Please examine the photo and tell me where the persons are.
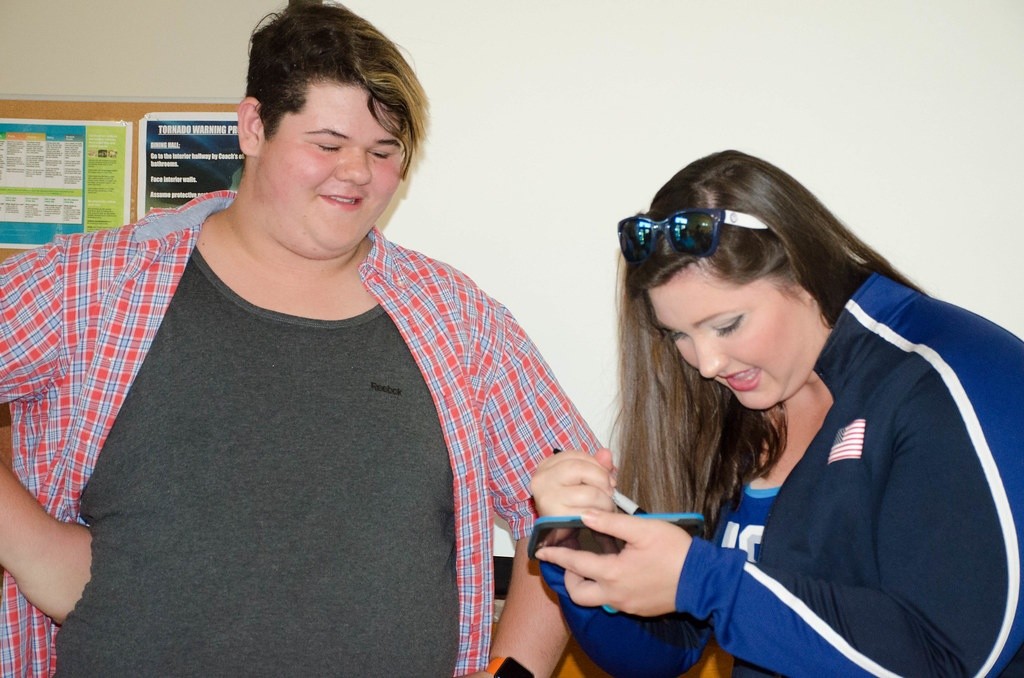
[531,151,1024,678]
[0,0,617,678]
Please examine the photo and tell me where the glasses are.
[618,210,769,263]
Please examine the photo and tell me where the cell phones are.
[526,512,704,562]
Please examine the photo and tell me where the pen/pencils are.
[551,448,647,515]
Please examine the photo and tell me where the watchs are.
[486,656,534,678]
